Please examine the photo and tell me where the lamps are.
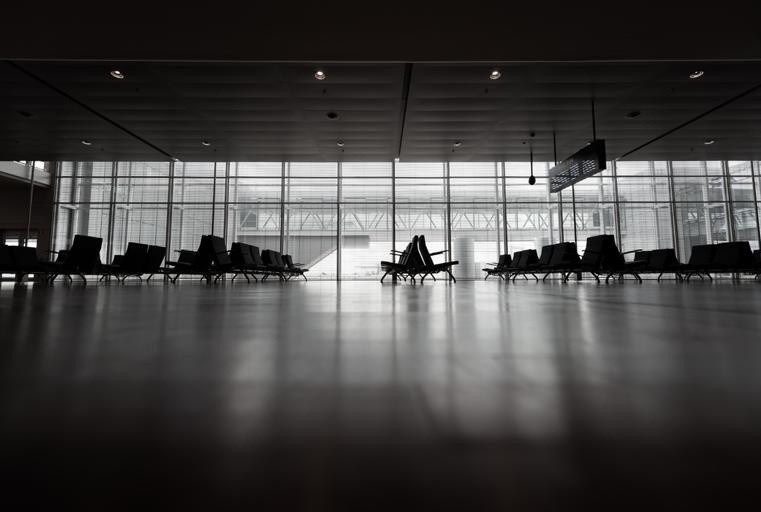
[312,67,328,81]
[687,62,705,79]
[703,132,720,145]
[486,65,505,83]
[109,63,128,81]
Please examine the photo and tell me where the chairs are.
[482,235,760,287]
[21,234,309,289]
[380,235,458,284]
